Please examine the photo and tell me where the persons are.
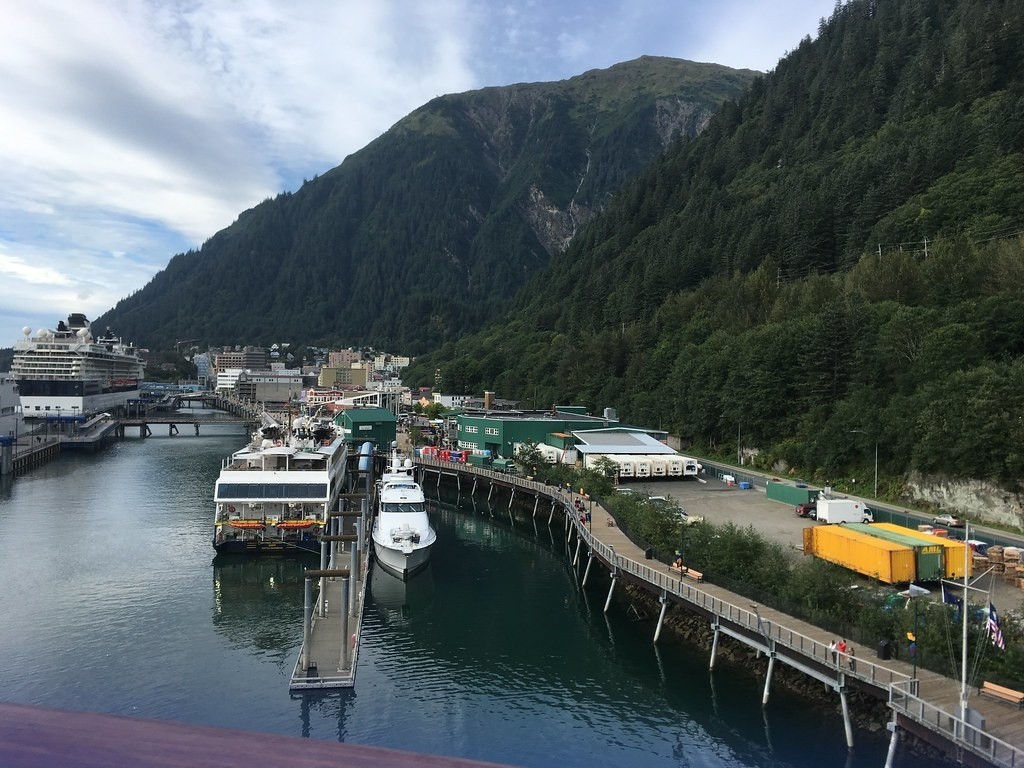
[574,496,585,525]
[829,640,837,664]
[837,639,846,664]
[847,647,855,671]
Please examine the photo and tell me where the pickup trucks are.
[796,503,818,520]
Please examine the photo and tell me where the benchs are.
[668,562,704,583]
[977,681,1024,711]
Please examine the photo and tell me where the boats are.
[211,408,350,560]
[370,558,438,621]
[110,379,125,387]
[371,439,437,574]
[126,380,138,387]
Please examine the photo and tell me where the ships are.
[8,313,148,419]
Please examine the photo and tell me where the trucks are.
[817,499,875,526]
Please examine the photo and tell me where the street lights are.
[850,430,878,499]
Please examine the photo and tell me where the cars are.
[933,514,965,528]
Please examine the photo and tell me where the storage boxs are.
[724,474,735,487]
[416,445,472,464]
[738,482,751,489]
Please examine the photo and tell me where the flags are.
[944,588,962,605]
[988,601,1005,650]
